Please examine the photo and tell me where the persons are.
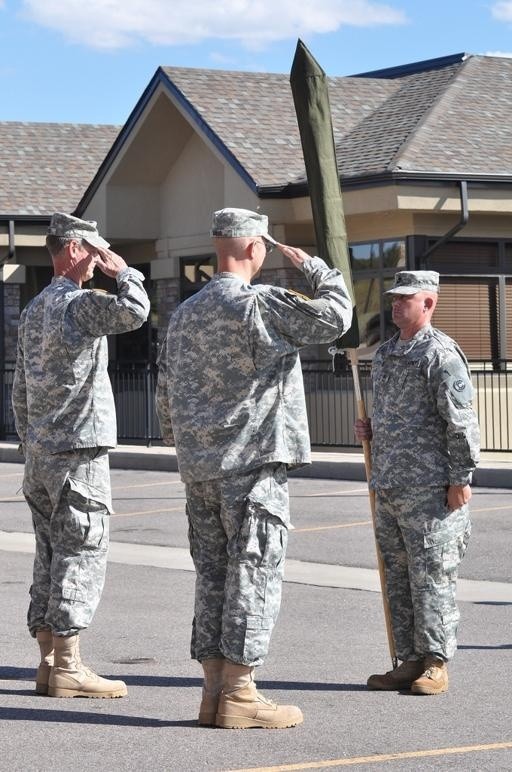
[153,207,355,732]
[354,270,481,694]
[11,211,150,699]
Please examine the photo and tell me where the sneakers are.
[367,661,424,690]
[412,660,448,694]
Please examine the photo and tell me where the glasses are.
[257,241,273,254]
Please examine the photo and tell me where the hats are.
[382,270,440,298]
[47,212,111,248]
[210,208,276,248]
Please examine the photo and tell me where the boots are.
[36,631,128,698]
[198,660,303,729]
